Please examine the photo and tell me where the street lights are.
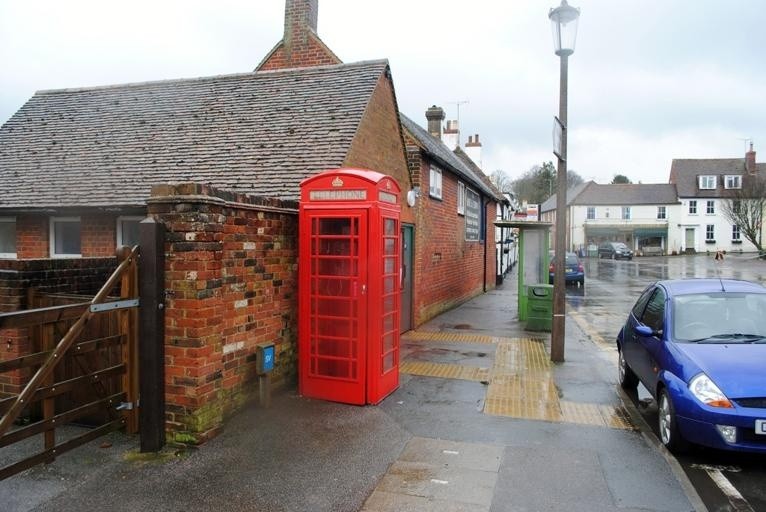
[457,100,471,148]
[544,1,586,364]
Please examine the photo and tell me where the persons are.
[715,250,726,261]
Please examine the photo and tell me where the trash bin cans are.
[524,284,554,332]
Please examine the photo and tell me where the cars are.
[614,267,765,468]
[543,249,586,288]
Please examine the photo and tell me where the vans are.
[595,240,635,261]
